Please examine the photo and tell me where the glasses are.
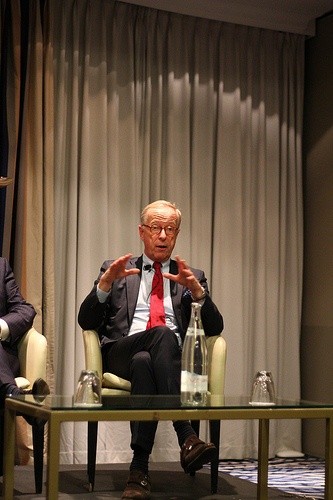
[142,223,178,235]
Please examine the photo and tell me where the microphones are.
[144,265,152,271]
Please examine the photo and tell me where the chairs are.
[14,326,49,494]
[82,328,227,496]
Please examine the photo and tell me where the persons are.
[0,258,51,426]
[78,200,223,500]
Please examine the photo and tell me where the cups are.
[73,369,103,408]
[249,370,277,407]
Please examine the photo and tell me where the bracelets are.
[191,286,206,301]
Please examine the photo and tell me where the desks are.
[0,395,333,500]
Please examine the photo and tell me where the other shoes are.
[21,378,50,425]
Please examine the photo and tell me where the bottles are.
[179,302,208,409]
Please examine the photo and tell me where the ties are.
[146,261,166,330]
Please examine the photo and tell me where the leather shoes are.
[122,470,149,500]
[179,436,216,475]
